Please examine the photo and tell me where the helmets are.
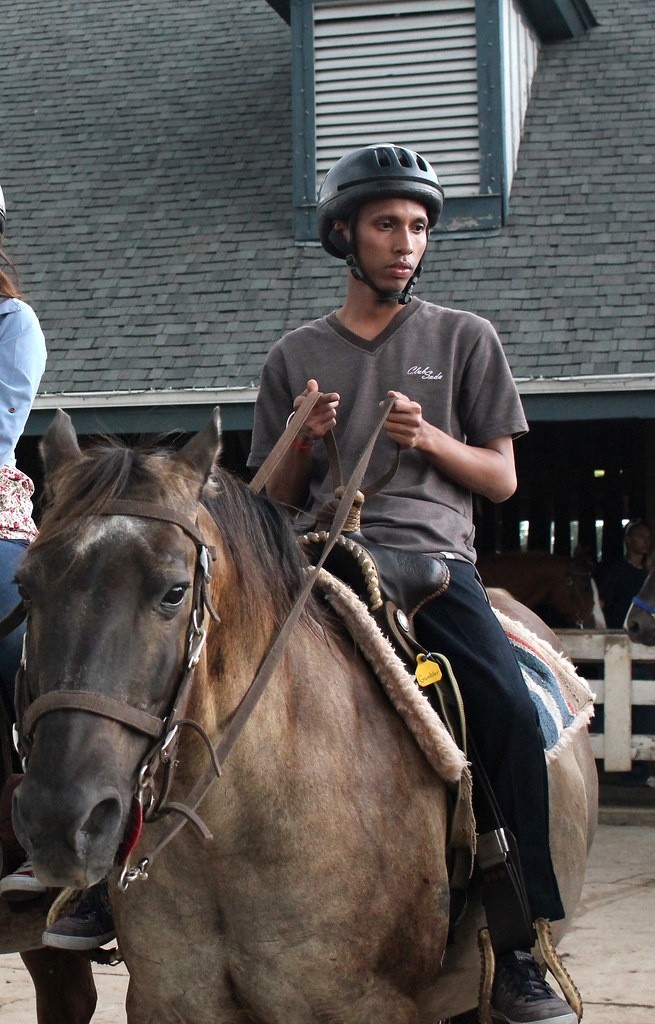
[314,143,446,260]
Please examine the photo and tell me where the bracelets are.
[284,411,317,451]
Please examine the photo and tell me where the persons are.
[43,144,580,1024]
[594,516,652,629]
[0,185,56,896]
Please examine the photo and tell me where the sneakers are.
[41,891,116,951]
[477,927,579,1024]
[0,852,47,902]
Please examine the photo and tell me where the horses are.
[621,559,654,649]
[0,404,608,1024]
